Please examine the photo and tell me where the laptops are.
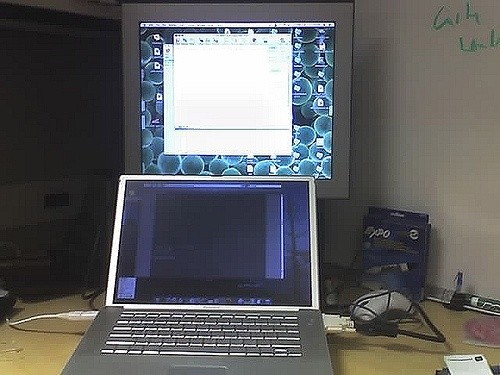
[60,173,333,375]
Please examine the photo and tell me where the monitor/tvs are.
[121,0,355,200]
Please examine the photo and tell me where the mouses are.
[348,288,414,332]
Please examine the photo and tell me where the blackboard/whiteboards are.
[353,2,500,324]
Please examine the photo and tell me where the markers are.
[459,294,500,313]
